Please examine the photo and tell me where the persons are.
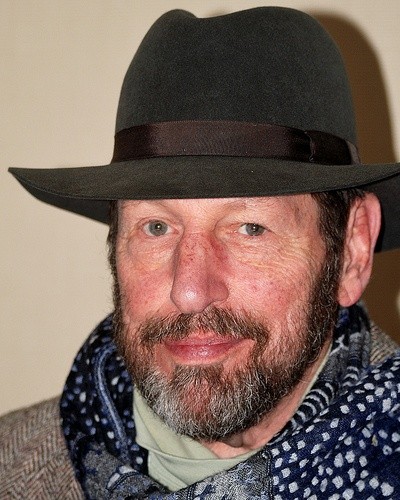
[0,6,399,499]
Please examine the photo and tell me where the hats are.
[8,9,400,254]
